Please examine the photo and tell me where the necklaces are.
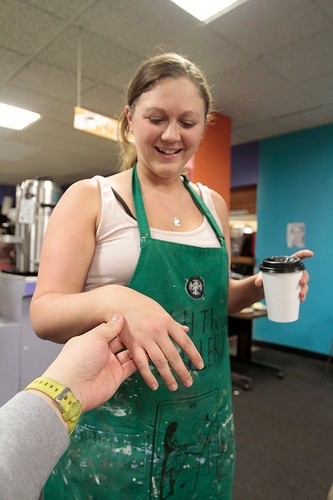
[145,182,187,227]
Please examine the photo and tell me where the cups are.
[259,256,305,323]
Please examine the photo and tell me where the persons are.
[28,51,314,500]
[0,312,149,500]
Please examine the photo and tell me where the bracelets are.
[25,377,81,438]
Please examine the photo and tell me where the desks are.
[228,308,285,392]
